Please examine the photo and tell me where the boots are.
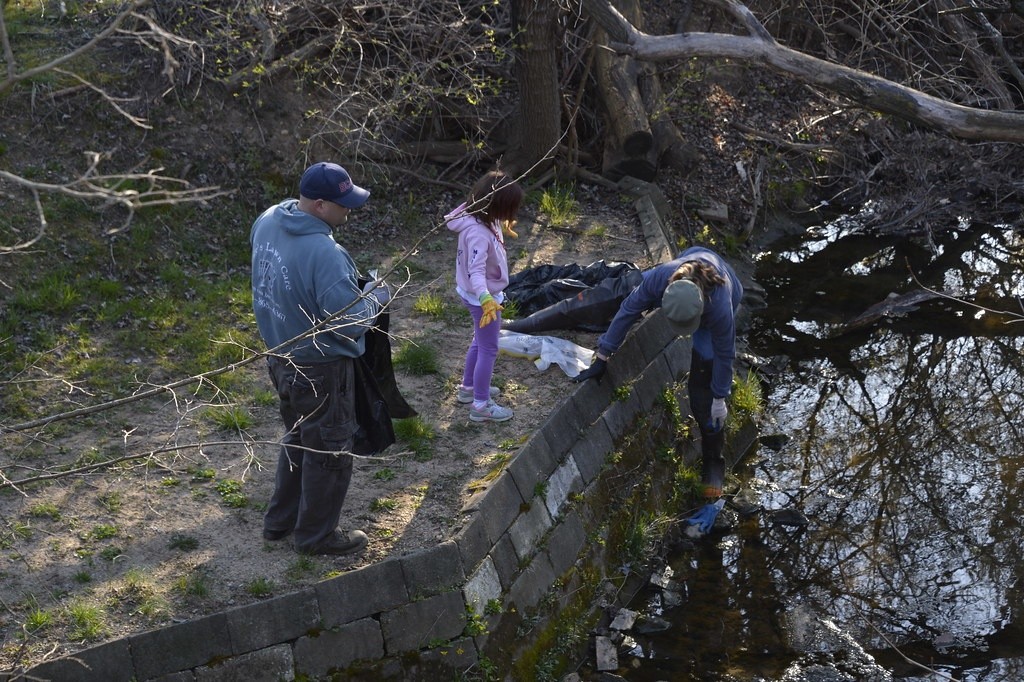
[687,347,726,497]
[501,269,644,334]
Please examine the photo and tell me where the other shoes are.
[295,527,369,555]
[263,527,292,540]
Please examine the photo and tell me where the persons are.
[501,246,744,498]
[249,162,392,555]
[443,170,523,422]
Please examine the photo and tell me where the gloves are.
[502,220,518,238]
[568,357,607,389]
[477,293,505,329]
[363,277,391,307]
[707,398,727,434]
[687,504,721,536]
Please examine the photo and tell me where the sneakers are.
[470,399,514,422]
[458,385,500,404]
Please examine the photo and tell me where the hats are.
[300,162,370,208]
[662,280,703,336]
